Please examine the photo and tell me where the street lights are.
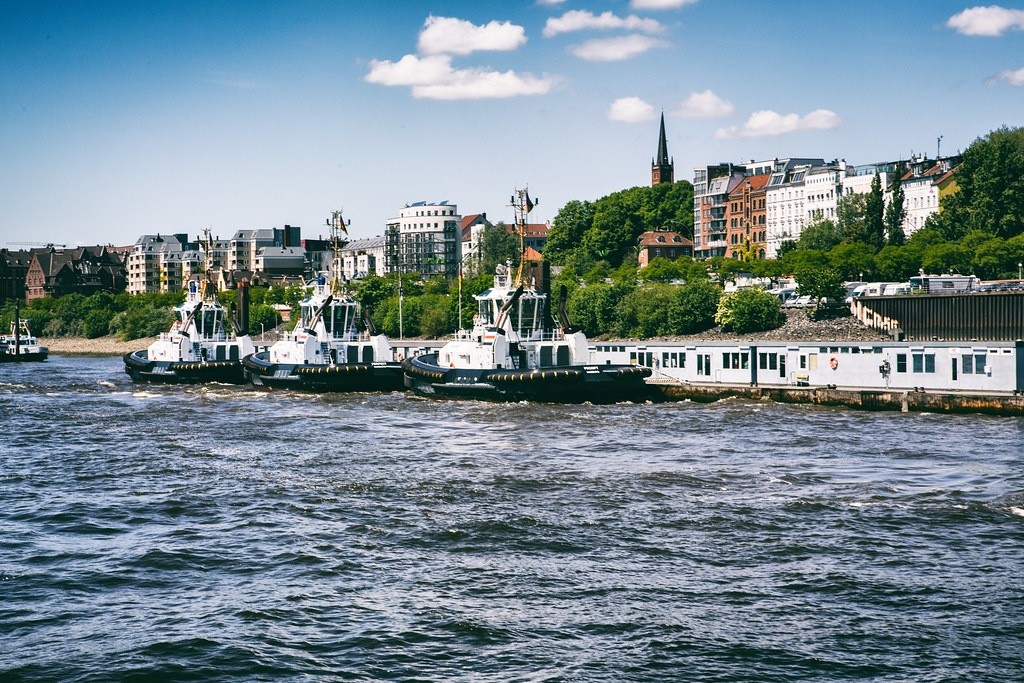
[1018,263,1022,281]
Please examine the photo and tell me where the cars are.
[727,276,1024,308]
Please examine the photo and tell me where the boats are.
[242,209,447,389]
[399,182,653,401]
[122,226,278,383]
[0,297,50,360]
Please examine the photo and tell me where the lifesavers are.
[830,357,839,370]
